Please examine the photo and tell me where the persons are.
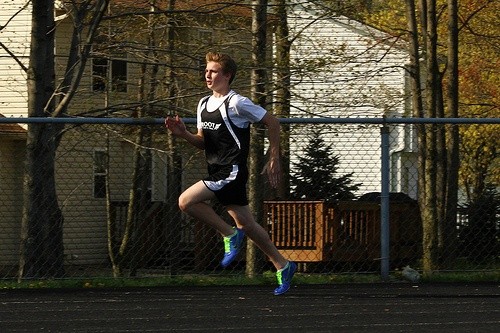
[165,51,298,296]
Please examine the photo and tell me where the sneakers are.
[273,262,297,295]
[221,226,244,268]
